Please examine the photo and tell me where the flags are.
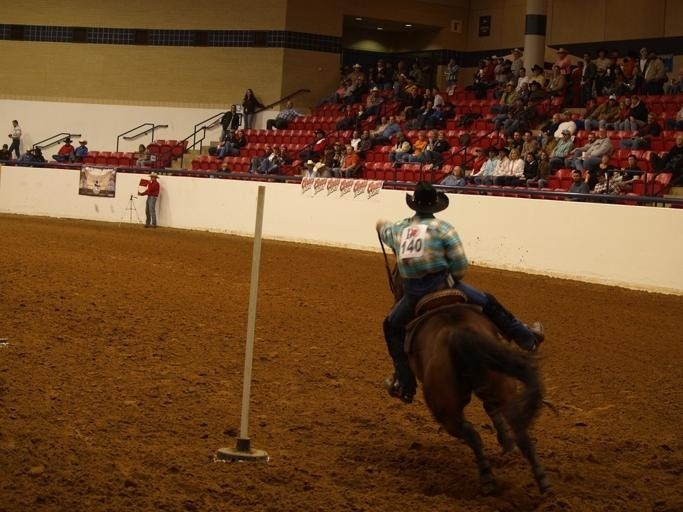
[139,178,149,188]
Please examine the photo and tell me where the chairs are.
[192,84,683,206]
[6,140,191,176]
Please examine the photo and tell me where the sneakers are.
[382,377,414,405]
[525,319,544,354]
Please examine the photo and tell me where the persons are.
[137,171,160,228]
[134,144,153,166]
[373,181,544,406]
[51,137,75,164]
[0,144,11,166]
[31,148,44,168]
[299,48,524,181]
[6,120,21,166]
[215,88,298,175]
[73,139,88,163]
[13,150,36,169]
[439,46,682,206]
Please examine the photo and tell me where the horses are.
[385,262,554,504]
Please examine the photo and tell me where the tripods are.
[118,195,143,228]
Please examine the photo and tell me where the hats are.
[405,180,450,216]
[147,172,160,179]
[64,138,72,143]
[78,139,87,145]
